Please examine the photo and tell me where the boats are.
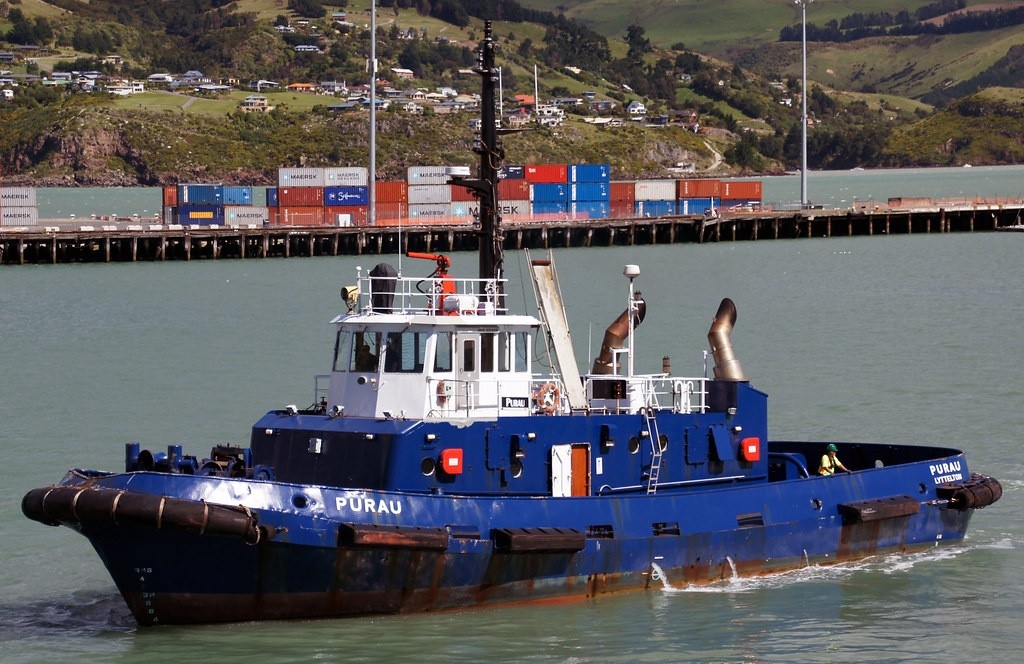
[20,10,1004,628]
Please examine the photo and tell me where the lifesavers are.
[536,383,560,414]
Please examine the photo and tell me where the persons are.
[817,443,852,477]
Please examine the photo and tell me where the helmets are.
[827,444,838,451]
